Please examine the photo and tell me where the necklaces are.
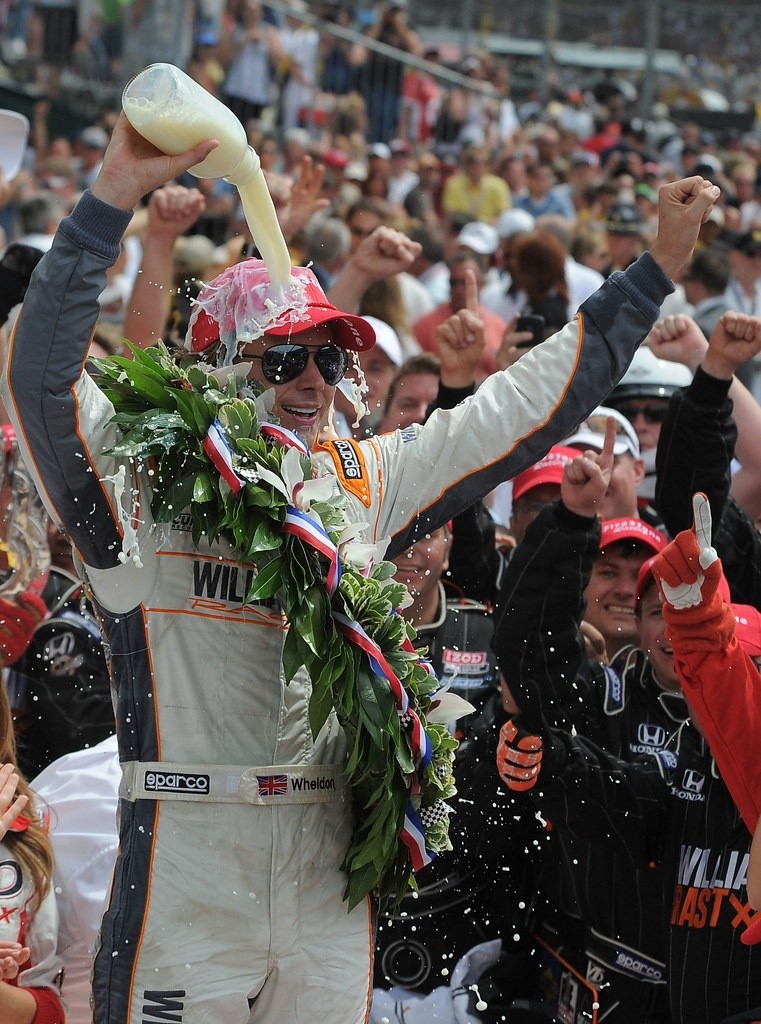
[92,347,477,915]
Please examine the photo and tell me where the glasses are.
[608,403,669,428]
[569,415,636,448]
[352,225,373,237]
[225,344,349,386]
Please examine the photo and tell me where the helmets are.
[602,346,696,399]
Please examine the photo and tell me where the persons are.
[0,97,721,1024]
[0,0,761,1024]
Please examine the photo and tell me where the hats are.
[599,517,667,557]
[556,407,641,463]
[185,259,376,357]
[634,552,731,614]
[731,603,761,657]
[512,445,589,499]
[359,315,402,369]
[497,209,537,241]
[454,222,497,255]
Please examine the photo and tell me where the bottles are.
[122,63,261,186]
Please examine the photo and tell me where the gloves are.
[496,678,567,794]
[651,491,722,612]
